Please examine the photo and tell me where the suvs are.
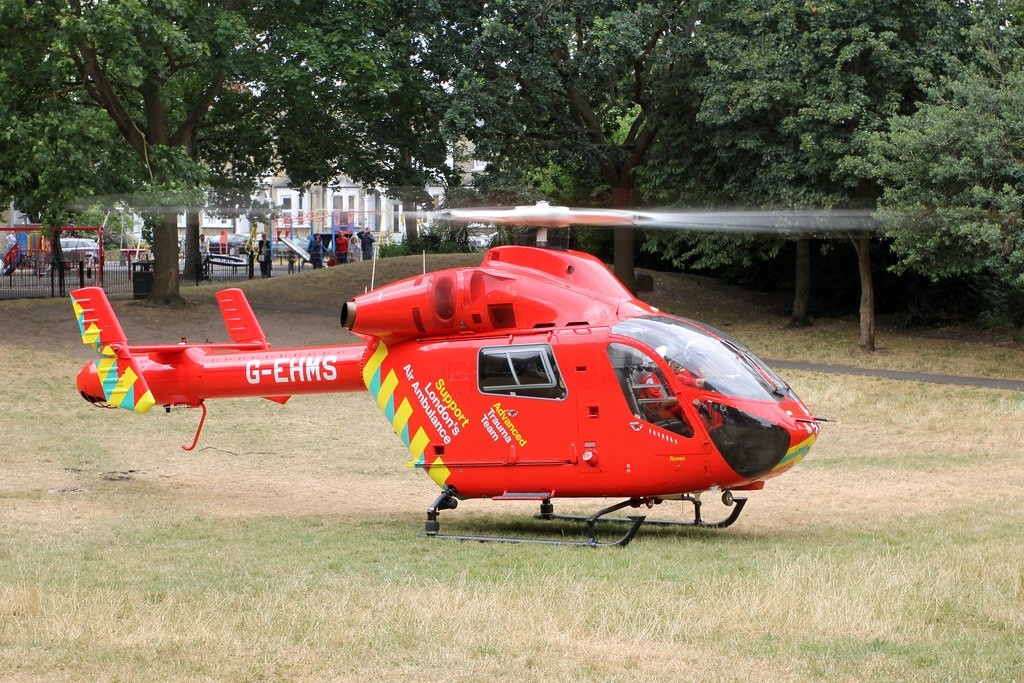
[60,238,104,267]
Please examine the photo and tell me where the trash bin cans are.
[130,259,155,300]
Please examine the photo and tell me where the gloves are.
[704,378,723,390]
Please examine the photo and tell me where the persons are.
[638,341,744,447]
[199,227,376,280]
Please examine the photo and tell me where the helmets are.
[685,342,704,362]
[655,345,673,368]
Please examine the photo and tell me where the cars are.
[210,234,251,257]
[252,232,310,255]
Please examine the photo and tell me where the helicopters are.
[67,200,938,549]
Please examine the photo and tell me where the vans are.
[463,223,501,250]
